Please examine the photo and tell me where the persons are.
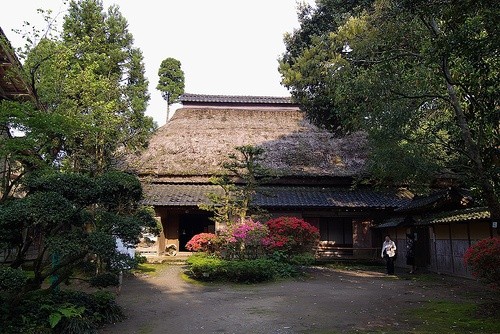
[405,233,418,275]
[380,235,397,275]
